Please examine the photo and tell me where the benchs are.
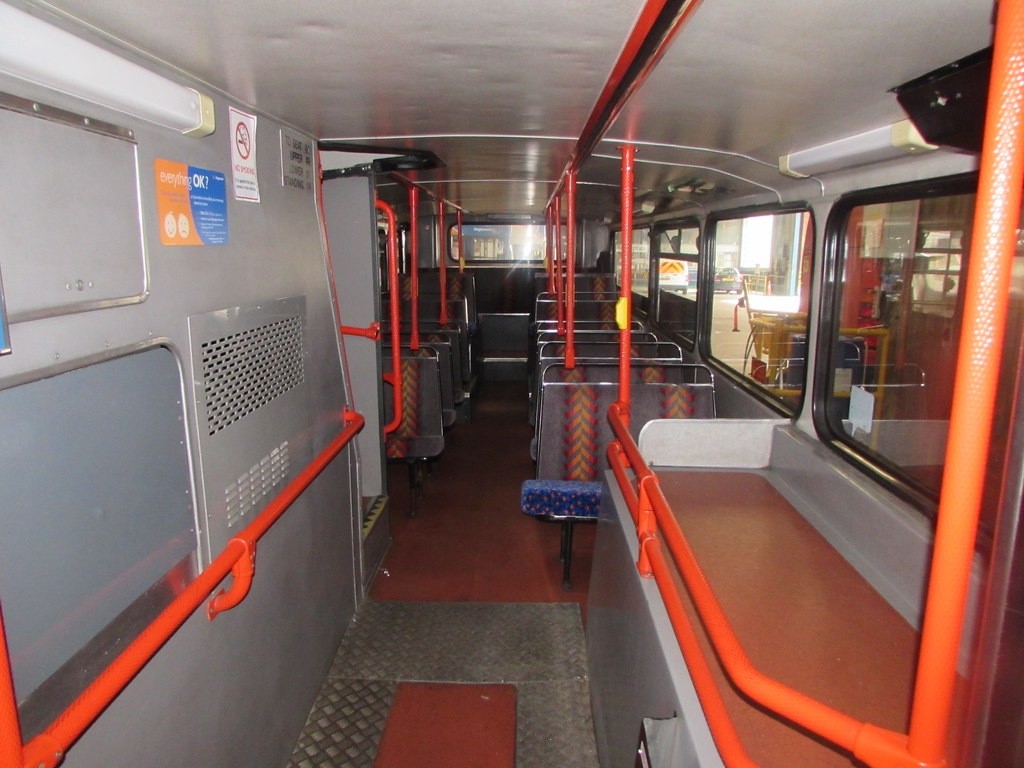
[381,268,719,592]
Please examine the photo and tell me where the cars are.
[713,267,743,294]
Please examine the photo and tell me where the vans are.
[658,258,689,294]
